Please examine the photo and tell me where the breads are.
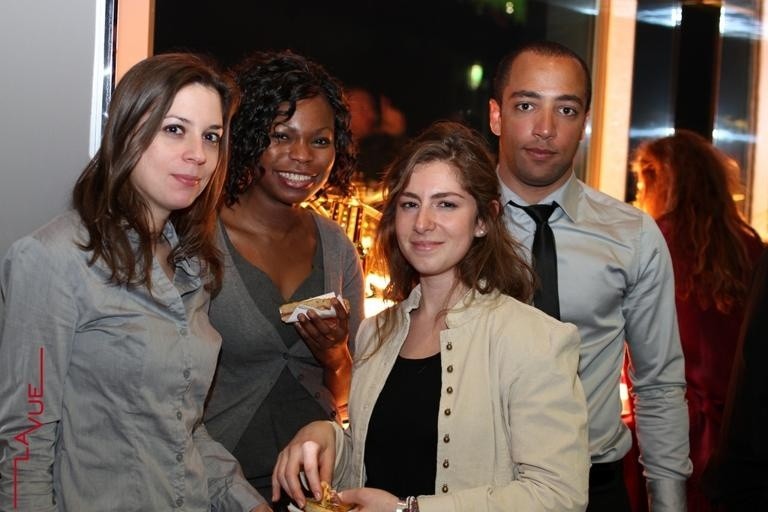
[302,480,357,512]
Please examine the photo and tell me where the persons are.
[621,126,767,512]
[205,51,368,512]
[477,39,697,512]
[0,50,273,511]
[270,118,593,512]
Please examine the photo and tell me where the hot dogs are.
[278,297,352,324]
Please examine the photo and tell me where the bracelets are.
[394,494,419,512]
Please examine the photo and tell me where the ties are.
[508,198,561,323]
